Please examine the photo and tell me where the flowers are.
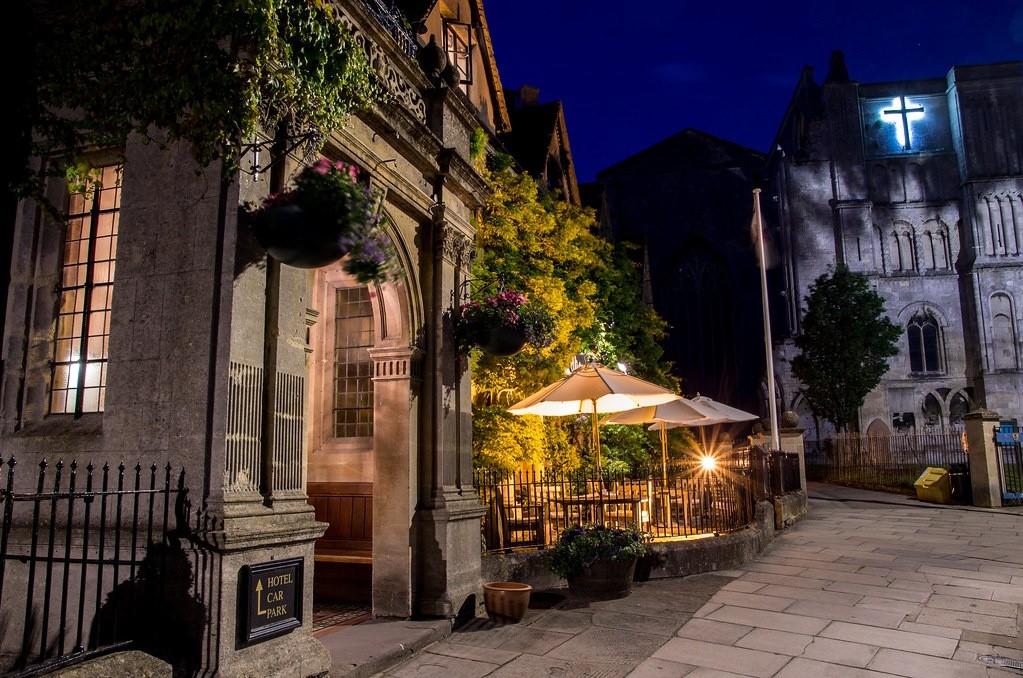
[541,522,647,602]
[247,159,407,287]
[451,289,554,358]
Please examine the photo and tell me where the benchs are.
[305,481,374,610]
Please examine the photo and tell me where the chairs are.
[495,488,544,554]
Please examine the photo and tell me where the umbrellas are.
[505,356,680,475]
[599,397,731,487]
[648,392,760,431]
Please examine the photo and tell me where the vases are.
[482,582,532,624]
[255,211,349,269]
[566,557,637,601]
[472,325,527,357]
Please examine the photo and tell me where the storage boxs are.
[914,467,952,504]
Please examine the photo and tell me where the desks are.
[550,497,641,530]
